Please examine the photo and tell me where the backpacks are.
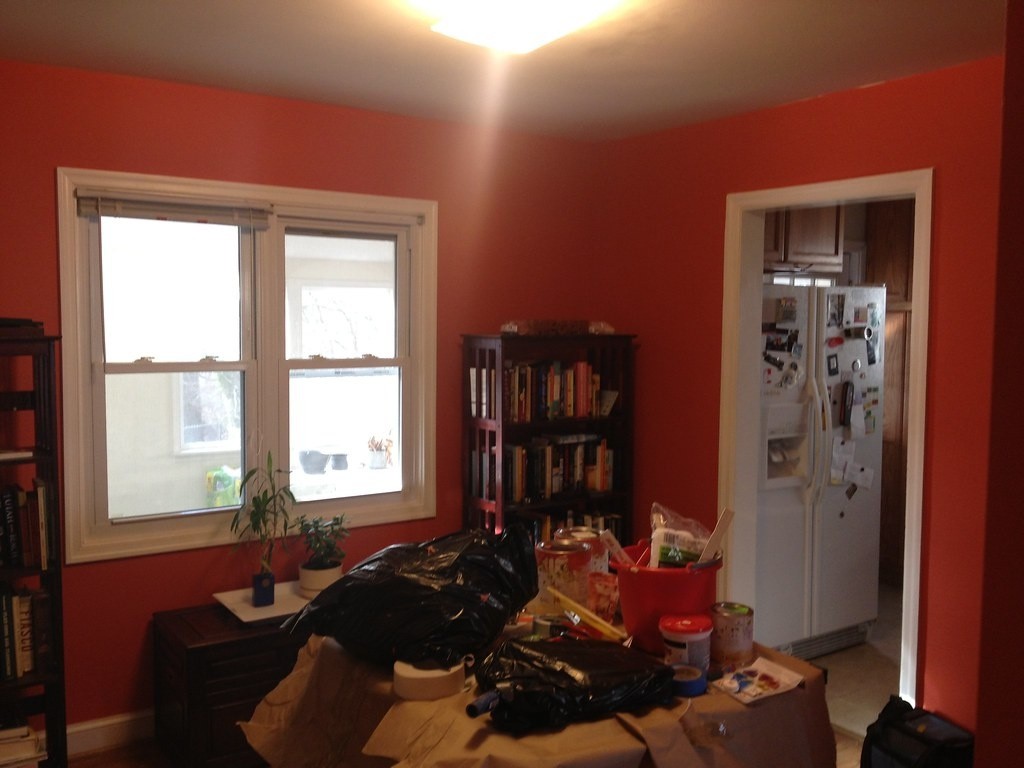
[860,695,976,768]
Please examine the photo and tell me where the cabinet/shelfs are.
[0,315,72,768]
[457,330,640,550]
[153,603,305,768]
[865,198,914,590]
[761,204,846,274]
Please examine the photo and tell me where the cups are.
[656,612,715,672]
[583,568,618,626]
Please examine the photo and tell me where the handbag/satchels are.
[266,520,541,678]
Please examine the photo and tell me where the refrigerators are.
[759,280,887,654]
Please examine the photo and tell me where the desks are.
[307,634,828,768]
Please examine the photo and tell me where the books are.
[600,390,619,416]
[470,357,622,551]
[0,478,47,571]
[1,592,62,680]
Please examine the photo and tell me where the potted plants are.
[287,513,351,598]
[230,450,299,607]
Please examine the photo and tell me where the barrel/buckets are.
[610,537,721,653]
[537,523,610,618]
